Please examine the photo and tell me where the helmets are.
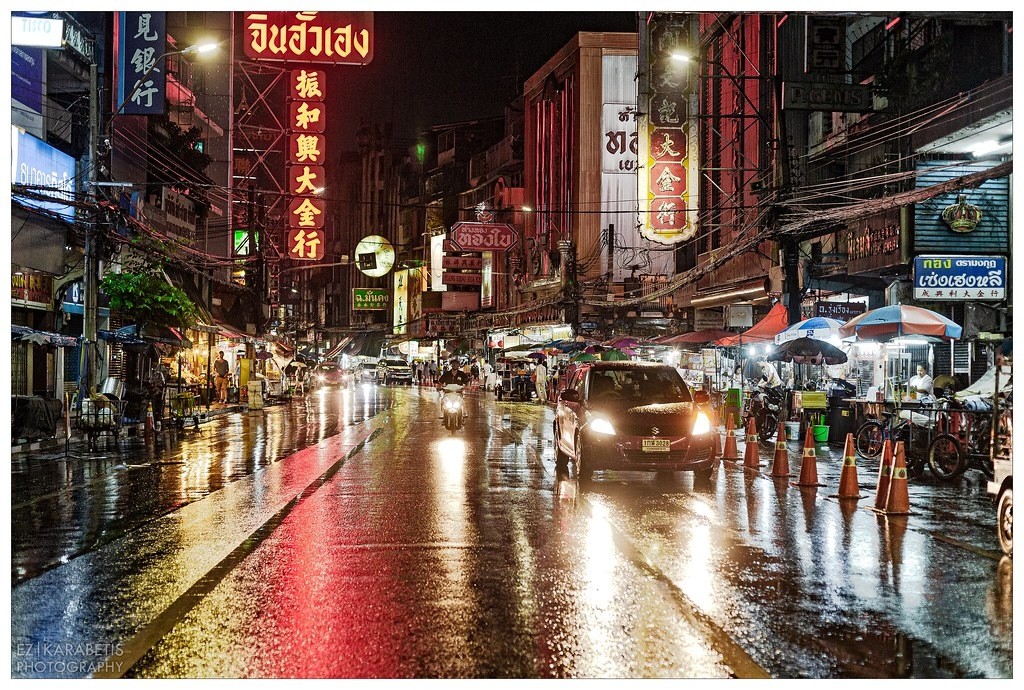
[448,355,460,366]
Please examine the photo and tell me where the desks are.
[11,395,62,444]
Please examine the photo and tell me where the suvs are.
[375,358,412,385]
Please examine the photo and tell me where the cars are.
[552,361,718,478]
[310,363,348,388]
[354,362,377,383]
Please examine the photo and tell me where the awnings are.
[11,324,78,346]
[498,343,545,352]
[162,265,296,356]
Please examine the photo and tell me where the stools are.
[70,393,77,412]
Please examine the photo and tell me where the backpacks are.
[297,369,303,382]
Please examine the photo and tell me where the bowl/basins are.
[828,396,851,407]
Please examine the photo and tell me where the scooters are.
[743,374,785,440]
[433,379,472,435]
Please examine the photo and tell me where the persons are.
[144,361,167,430]
[294,364,304,398]
[732,365,748,387]
[534,359,548,405]
[471,361,479,390]
[905,361,933,399]
[756,356,786,424]
[411,360,438,386]
[213,351,229,404]
[481,360,494,391]
[438,359,470,426]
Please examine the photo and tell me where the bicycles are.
[854,399,995,479]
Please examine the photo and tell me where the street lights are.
[522,207,578,336]
[255,186,324,335]
[670,52,803,326]
[82,37,218,393]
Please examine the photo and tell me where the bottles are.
[876,384,917,401]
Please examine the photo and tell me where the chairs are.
[597,376,616,400]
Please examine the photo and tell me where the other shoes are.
[224,399,228,404]
[219,399,222,403]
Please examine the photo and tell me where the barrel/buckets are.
[99,377,123,400]
[813,425,830,441]
[784,421,800,440]
[825,407,855,442]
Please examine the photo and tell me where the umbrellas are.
[839,301,963,407]
[644,327,745,389]
[766,335,848,382]
[94,322,193,382]
[525,336,644,361]
[774,314,855,344]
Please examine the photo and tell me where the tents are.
[712,301,809,389]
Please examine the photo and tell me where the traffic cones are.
[415,375,446,387]
[827,433,870,499]
[863,439,919,517]
[763,422,796,477]
[713,411,743,460]
[470,376,480,391]
[738,418,766,468]
[788,428,828,487]
[548,383,560,402]
[142,398,157,433]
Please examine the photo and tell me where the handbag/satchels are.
[530,374,536,382]
